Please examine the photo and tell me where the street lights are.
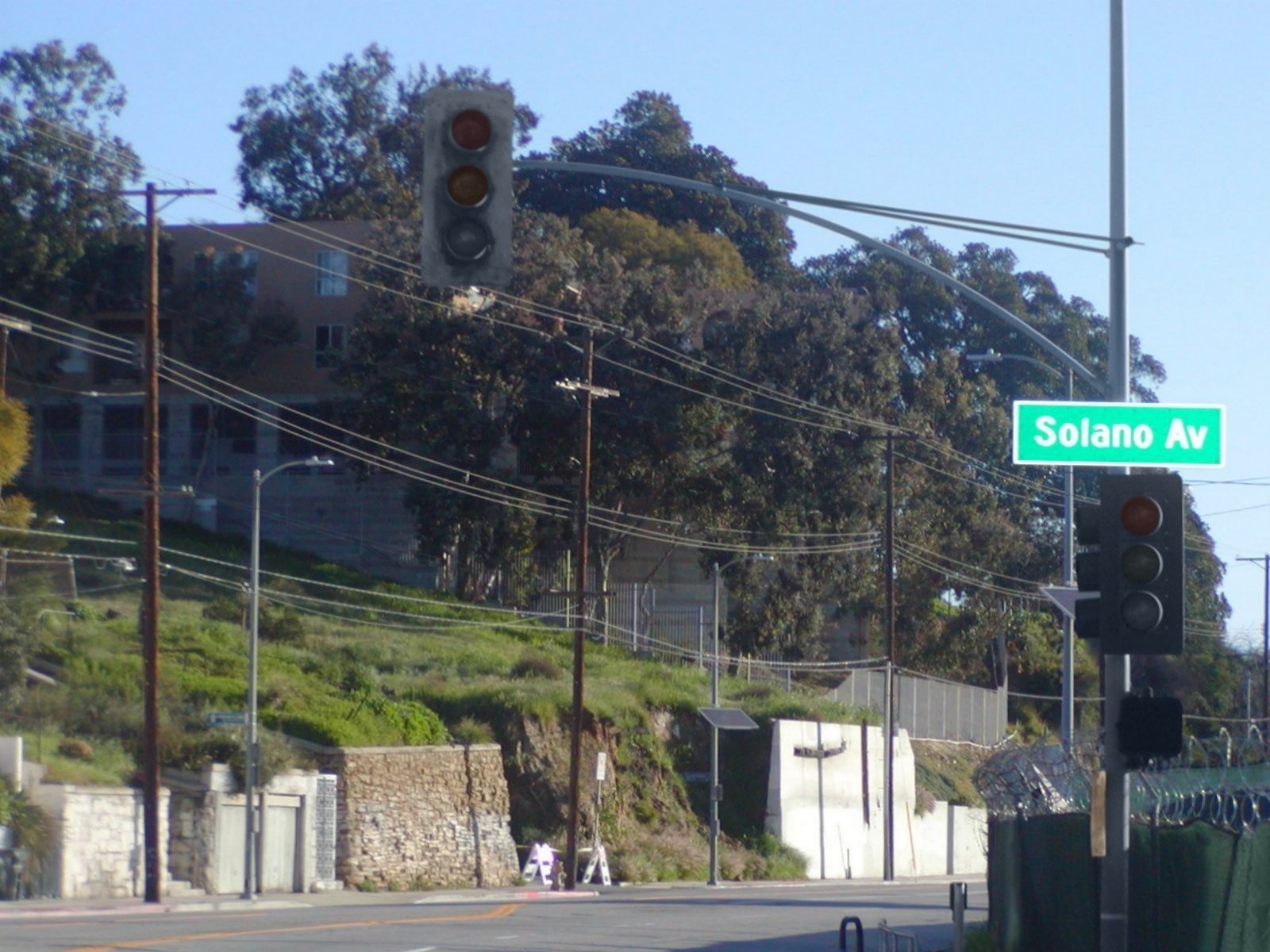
[238,454,336,902]
[695,554,775,889]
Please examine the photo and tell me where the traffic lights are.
[1072,504,1102,641]
[1091,469,1185,653]
[1119,692,1184,760]
[422,84,512,291]
